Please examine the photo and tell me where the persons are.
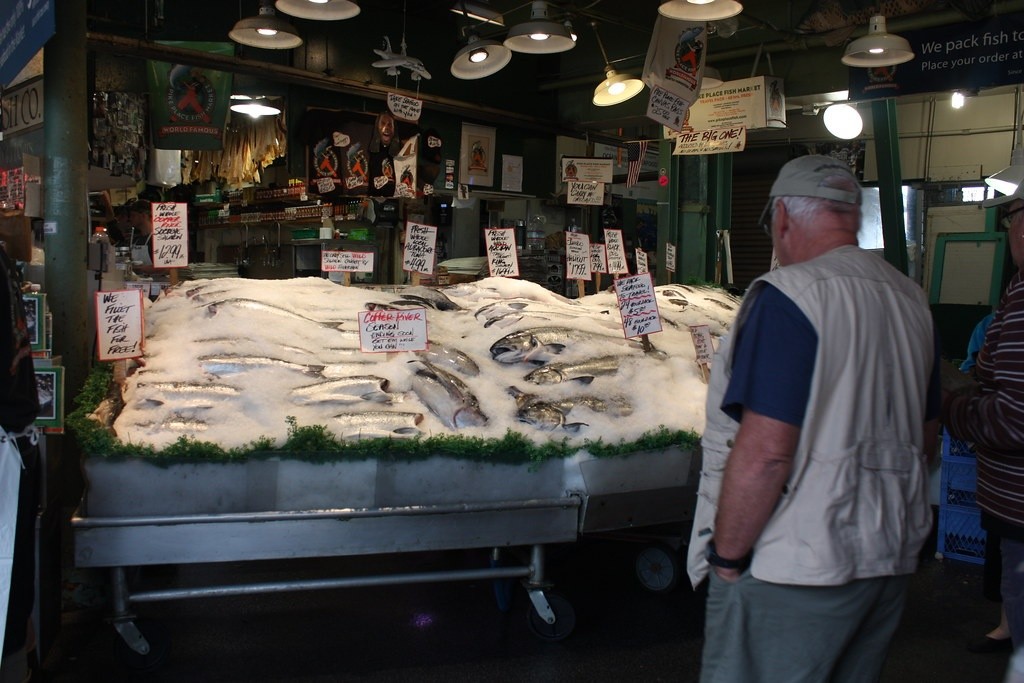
[102,189,169,277]
[943,178,1024,683]
[378,113,395,145]
[687,155,941,683]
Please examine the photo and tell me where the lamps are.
[841,0,916,67]
[700,65,724,90]
[450,0,512,80]
[823,103,863,139]
[503,0,576,54]
[589,19,645,106]
[230,75,282,119]
[228,0,304,50]
[657,0,744,21]
[274,0,361,20]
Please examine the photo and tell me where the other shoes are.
[968,634,1013,653]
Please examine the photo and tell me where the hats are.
[982,179,1024,209]
[758,155,862,227]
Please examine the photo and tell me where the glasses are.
[762,206,777,238]
[999,207,1024,227]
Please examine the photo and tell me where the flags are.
[626,141,648,189]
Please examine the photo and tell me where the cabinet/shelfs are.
[189,195,414,286]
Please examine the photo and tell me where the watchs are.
[705,540,753,570]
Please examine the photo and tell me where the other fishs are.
[129,275,742,445]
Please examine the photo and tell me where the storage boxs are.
[937,424,986,564]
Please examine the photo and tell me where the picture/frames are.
[458,122,497,186]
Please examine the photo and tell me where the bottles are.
[323,200,364,216]
[31,284,42,294]
[216,187,222,203]
[287,179,305,195]
[91,227,109,244]
